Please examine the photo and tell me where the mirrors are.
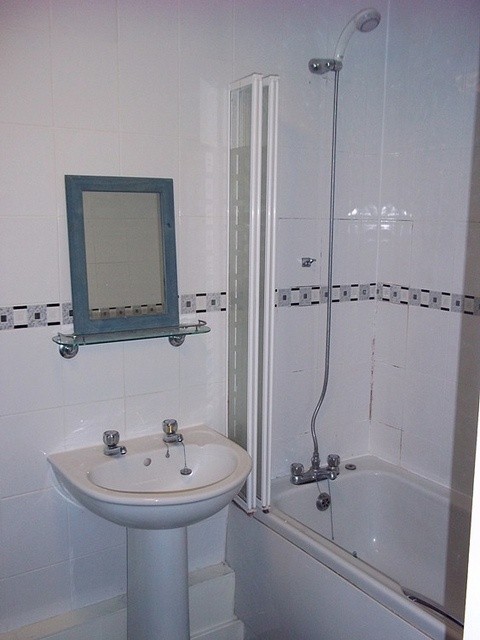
[65,174,179,335]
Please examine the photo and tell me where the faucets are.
[160,419,182,443]
[102,429,127,457]
[310,465,338,483]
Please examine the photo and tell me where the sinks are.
[44,424,254,505]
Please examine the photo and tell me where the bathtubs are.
[236,451,473,640]
